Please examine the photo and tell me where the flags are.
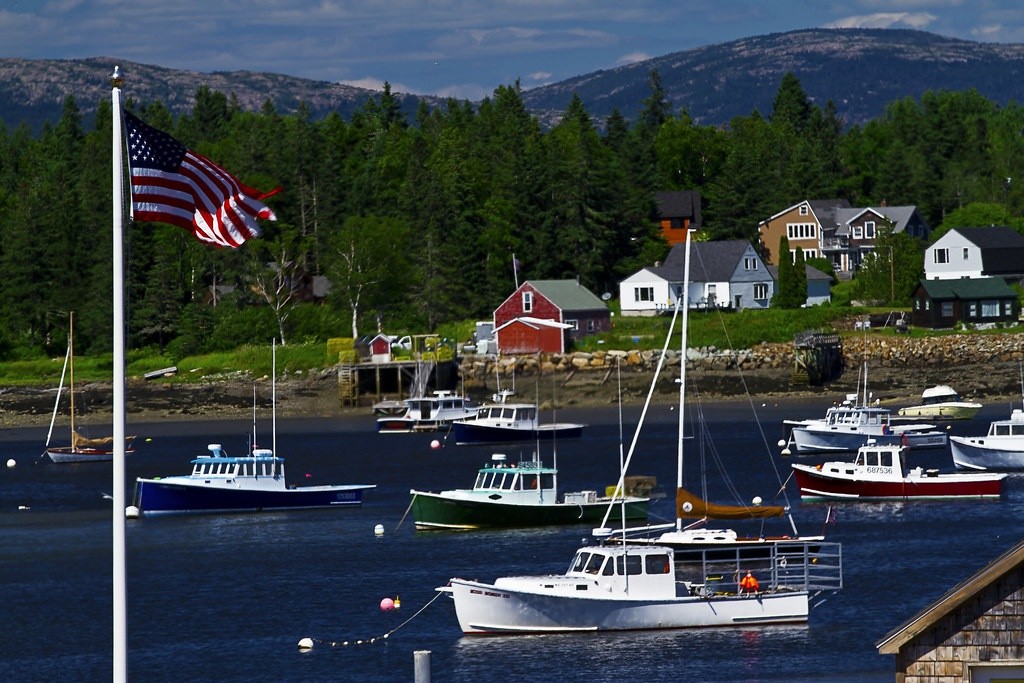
[120,109,282,250]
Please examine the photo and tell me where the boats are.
[451,350,591,448]
[896,382,984,423]
[790,387,1011,503]
[948,360,1024,475]
[371,345,491,437]
[409,364,670,532]
[128,336,381,520]
[783,322,950,457]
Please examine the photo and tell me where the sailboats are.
[435,225,844,641]
[43,310,138,465]
